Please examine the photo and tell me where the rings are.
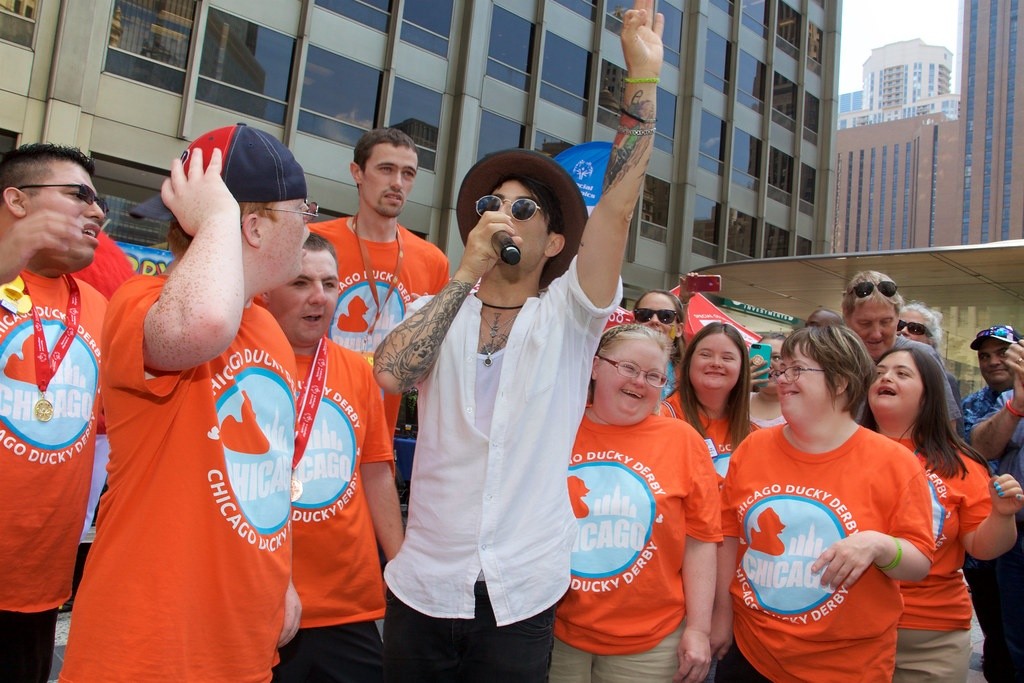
[1016,357,1023,364]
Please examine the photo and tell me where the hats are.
[970,325,1020,350]
[455,149,588,288]
[128,123,308,222]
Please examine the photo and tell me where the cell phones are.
[748,344,772,386]
[686,275,721,292]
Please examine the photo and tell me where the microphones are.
[491,230,521,265]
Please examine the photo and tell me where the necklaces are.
[479,319,513,367]
[474,291,525,309]
[479,311,513,338]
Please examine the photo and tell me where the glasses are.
[849,281,898,298]
[768,366,824,384]
[976,326,1019,340]
[598,355,669,388]
[262,201,319,222]
[897,319,933,338]
[475,195,547,222]
[18,184,109,219]
[633,308,681,324]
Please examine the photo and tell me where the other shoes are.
[58,601,73,612]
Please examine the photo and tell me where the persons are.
[0,0,1024,683]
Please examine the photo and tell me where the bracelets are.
[624,76,662,86]
[1005,400,1023,419]
[879,536,903,571]
[617,124,658,138]
[620,107,658,125]
